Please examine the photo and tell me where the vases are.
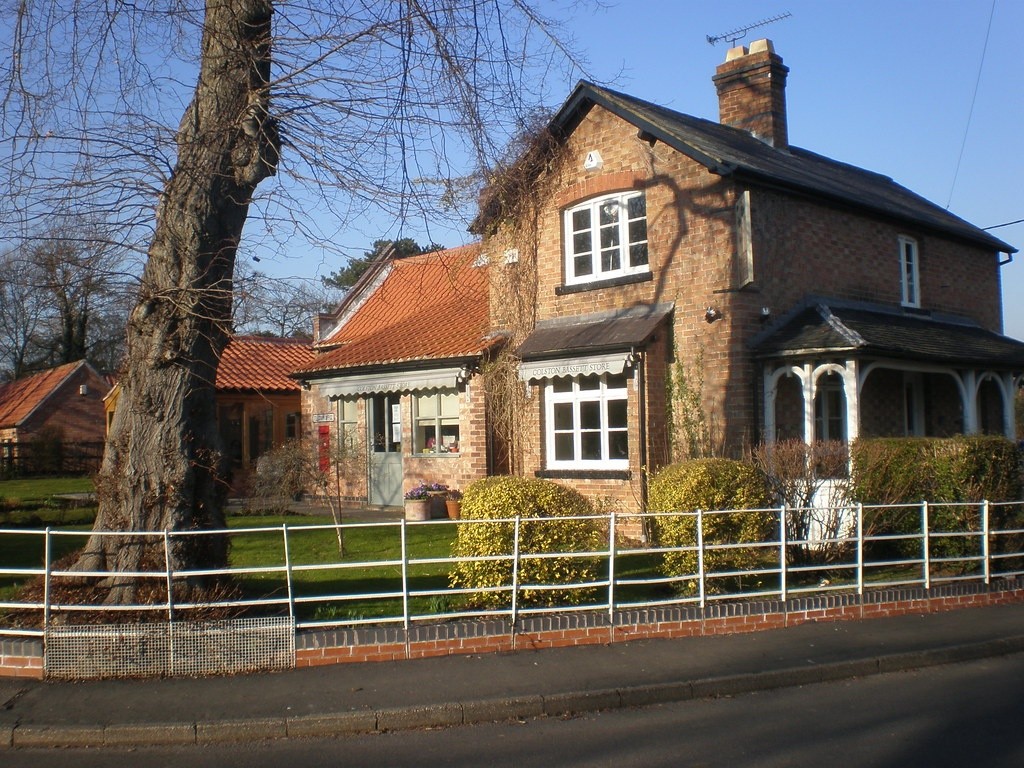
[427,491,449,519]
[404,499,431,521]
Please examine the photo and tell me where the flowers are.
[404,484,432,499]
[427,483,449,491]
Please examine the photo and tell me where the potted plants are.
[445,489,463,519]
